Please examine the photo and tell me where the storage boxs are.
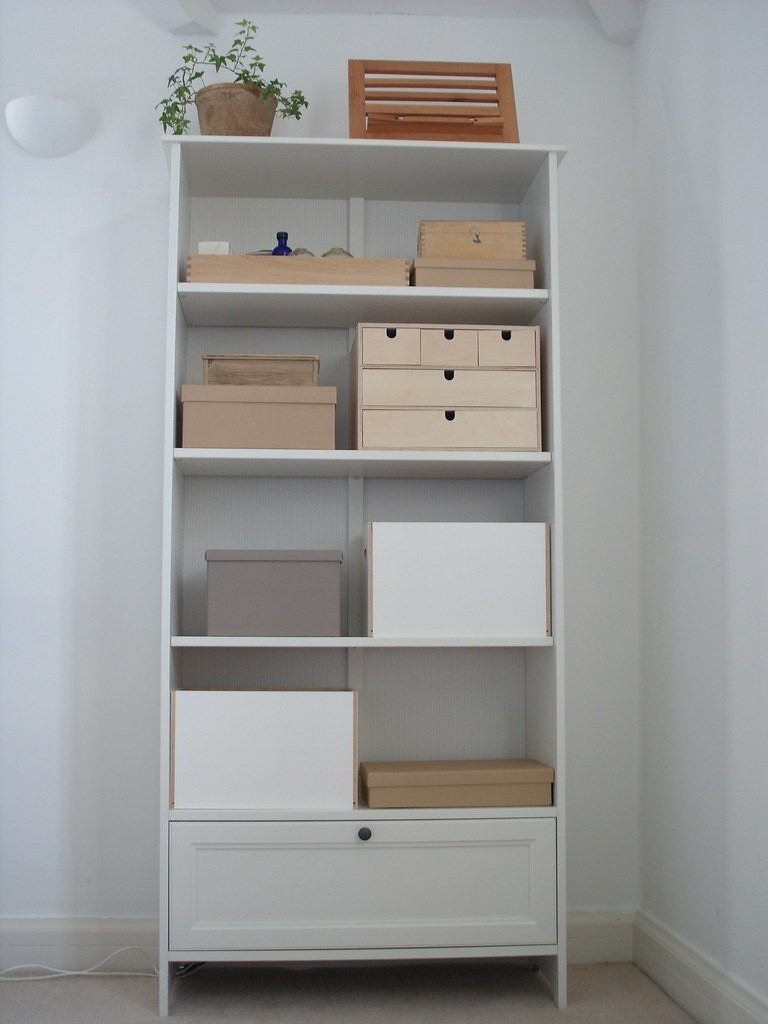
[181,385,337,449]
[363,521,551,638]
[408,257,534,290]
[169,688,359,809]
[361,758,554,806]
[417,219,527,261]
[203,354,320,385]
[205,548,343,635]
[186,256,410,287]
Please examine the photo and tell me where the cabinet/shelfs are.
[158,135,570,1016]
[350,324,543,452]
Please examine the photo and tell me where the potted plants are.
[155,20,310,137]
